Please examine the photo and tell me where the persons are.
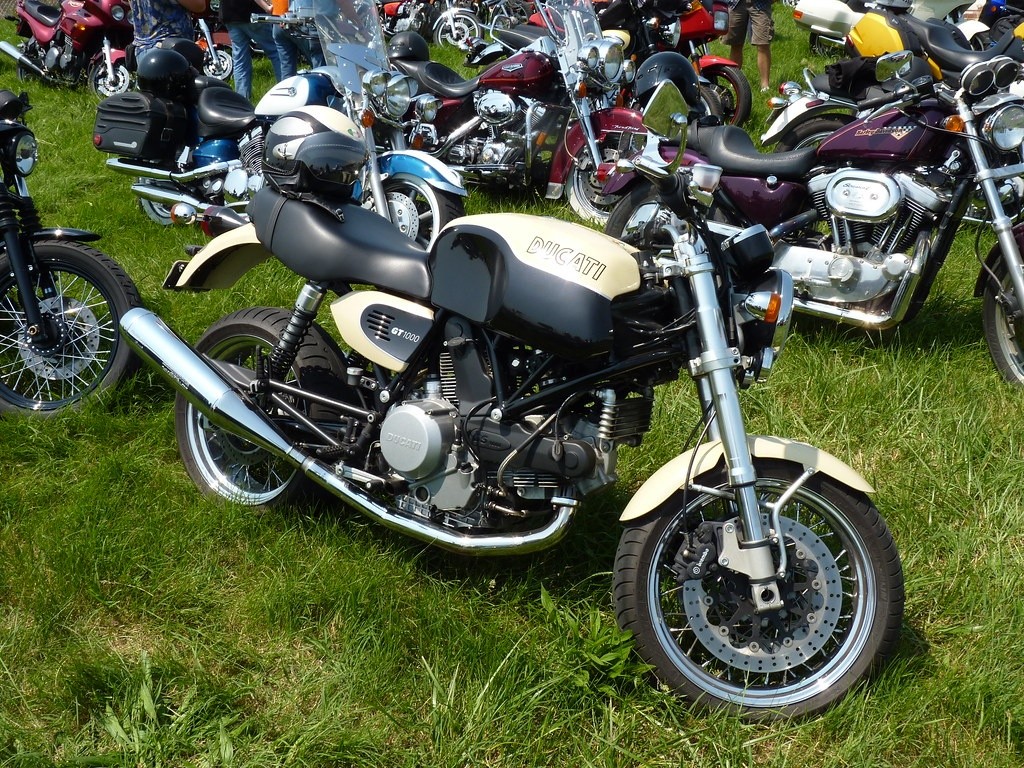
[128,0,206,71]
[718,0,778,93]
[220,0,281,101]
[272,0,369,83]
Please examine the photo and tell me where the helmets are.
[261,105,370,223]
[137,48,200,99]
[634,51,706,113]
[387,31,429,61]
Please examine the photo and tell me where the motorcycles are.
[92,0,468,247]
[0,0,751,229]
[603,1,1024,386]
[118,79,905,726]
[0,90,145,422]
